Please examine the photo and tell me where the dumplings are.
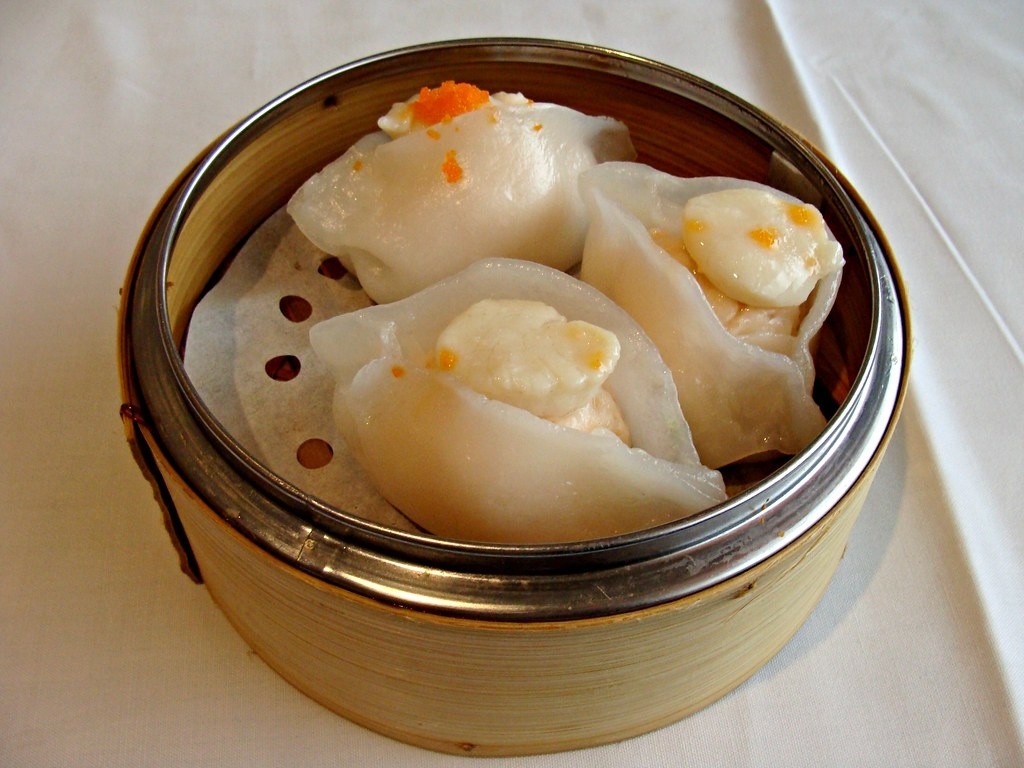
[283,80,847,545]
